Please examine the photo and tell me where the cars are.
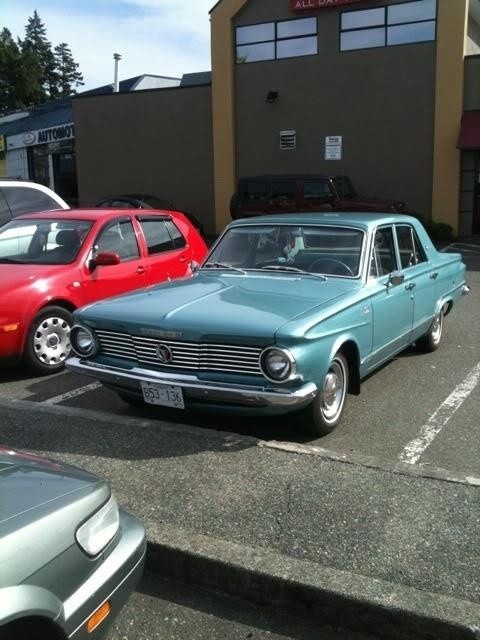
[0,445,151,639]
[62,207,471,437]
[93,192,201,228]
[0,204,211,375]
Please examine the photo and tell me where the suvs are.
[0,174,73,259]
[229,173,409,222]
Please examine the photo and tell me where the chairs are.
[254,247,415,279]
[46,230,123,263]
